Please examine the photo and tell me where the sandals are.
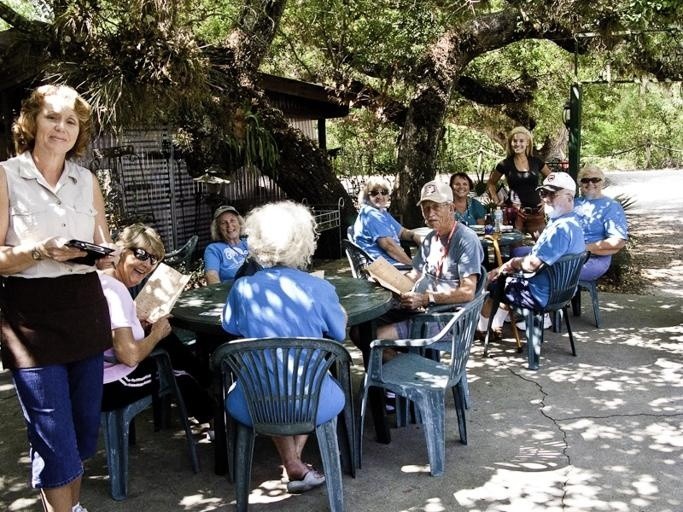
[385,397,396,413]
[494,328,502,339]
[476,329,489,341]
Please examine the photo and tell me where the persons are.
[353,176,424,282]
[511,166,629,280]
[449,174,485,228]
[373,181,483,414]
[0,85,122,511]
[204,205,247,286]
[487,125,551,246]
[221,202,351,490]
[98,223,224,445]
[475,172,585,341]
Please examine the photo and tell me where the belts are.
[522,207,538,212]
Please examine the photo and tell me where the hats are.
[212,205,240,220]
[416,179,453,207]
[535,172,577,197]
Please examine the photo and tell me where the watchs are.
[410,234,416,241]
[31,244,45,261]
[428,293,435,306]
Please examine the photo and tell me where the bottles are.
[494,206,504,233]
[484,214,493,234]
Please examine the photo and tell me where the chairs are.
[346,224,413,272]
[342,238,376,283]
[483,250,592,370]
[357,290,490,477]
[409,266,486,424]
[100,351,202,501]
[210,336,359,512]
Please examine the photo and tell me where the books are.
[133,263,196,327]
[362,256,415,298]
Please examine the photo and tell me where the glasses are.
[539,190,568,198]
[129,247,160,266]
[367,189,388,196]
[581,178,603,183]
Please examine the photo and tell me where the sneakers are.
[208,429,215,440]
[71,502,88,512]
[544,309,564,329]
[516,314,544,330]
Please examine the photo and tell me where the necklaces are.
[227,239,250,259]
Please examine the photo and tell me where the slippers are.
[281,464,319,483]
[288,470,326,493]
